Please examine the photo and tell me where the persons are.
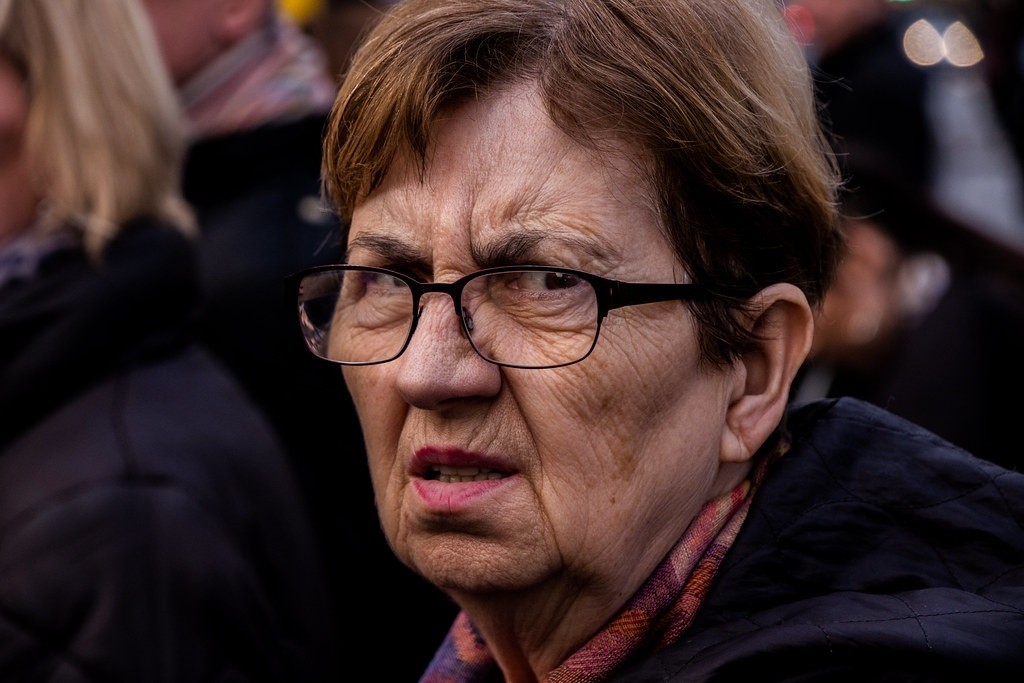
[0,0,466,683]
[322,0,1024,683]
[793,0,1024,474]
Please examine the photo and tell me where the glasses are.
[284,265,750,369]
